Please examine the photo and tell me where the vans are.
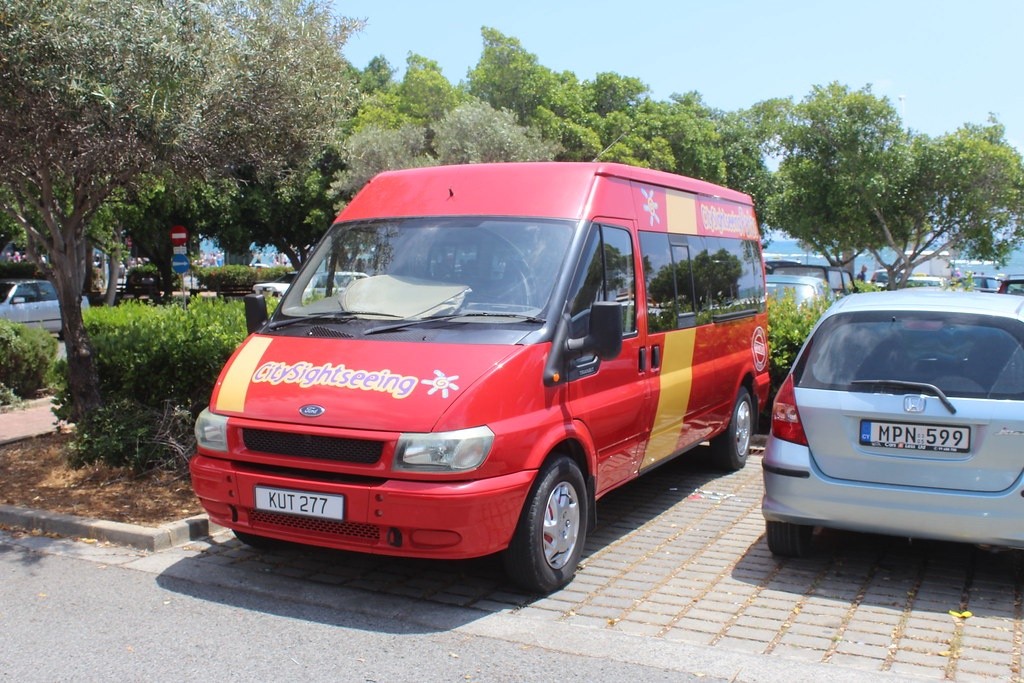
[188,161,771,596]
[763,261,859,303]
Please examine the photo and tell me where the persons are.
[952,267,988,288]
[1,248,292,289]
[857,264,868,282]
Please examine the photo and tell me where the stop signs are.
[169,225,187,246]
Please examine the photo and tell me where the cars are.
[253,270,371,305]
[763,274,836,319]
[868,266,1024,297]
[762,290,1024,559]
[0,278,91,341]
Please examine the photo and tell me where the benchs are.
[115,284,166,304]
[189,288,251,298]
